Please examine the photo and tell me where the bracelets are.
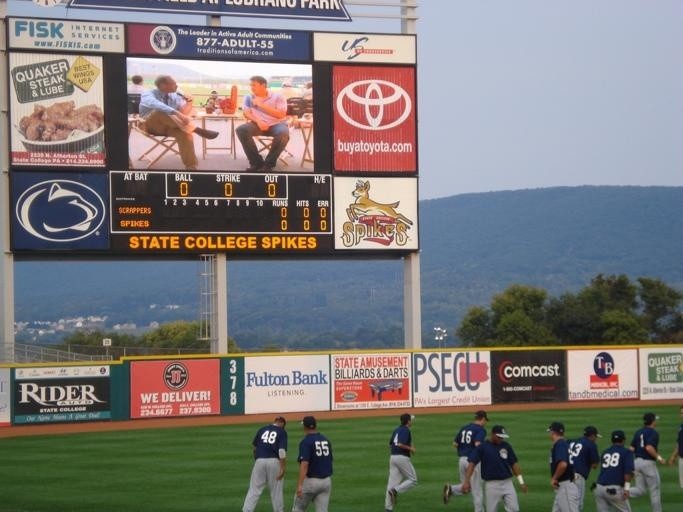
[516,475,524,485]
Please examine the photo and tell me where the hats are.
[643,413,659,421]
[401,414,415,420]
[584,426,604,438]
[303,416,316,429]
[476,410,489,421]
[492,426,510,439]
[612,430,624,440]
[276,416,285,428]
[547,422,565,434]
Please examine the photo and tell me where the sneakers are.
[389,489,397,504]
[443,484,450,503]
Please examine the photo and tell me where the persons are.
[290,416,333,512]
[384,413,418,512]
[235,76,289,173]
[463,424,528,512]
[240,416,288,512]
[544,404,683,512]
[444,410,489,512]
[138,75,219,169]
[130,76,148,94]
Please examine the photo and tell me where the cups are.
[215,109,223,114]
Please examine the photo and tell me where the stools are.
[135,126,182,169]
[255,134,293,165]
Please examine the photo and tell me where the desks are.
[295,118,313,167]
[197,111,242,160]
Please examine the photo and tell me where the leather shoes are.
[199,130,219,139]
[247,164,265,170]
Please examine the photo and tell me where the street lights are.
[434,327,446,349]
